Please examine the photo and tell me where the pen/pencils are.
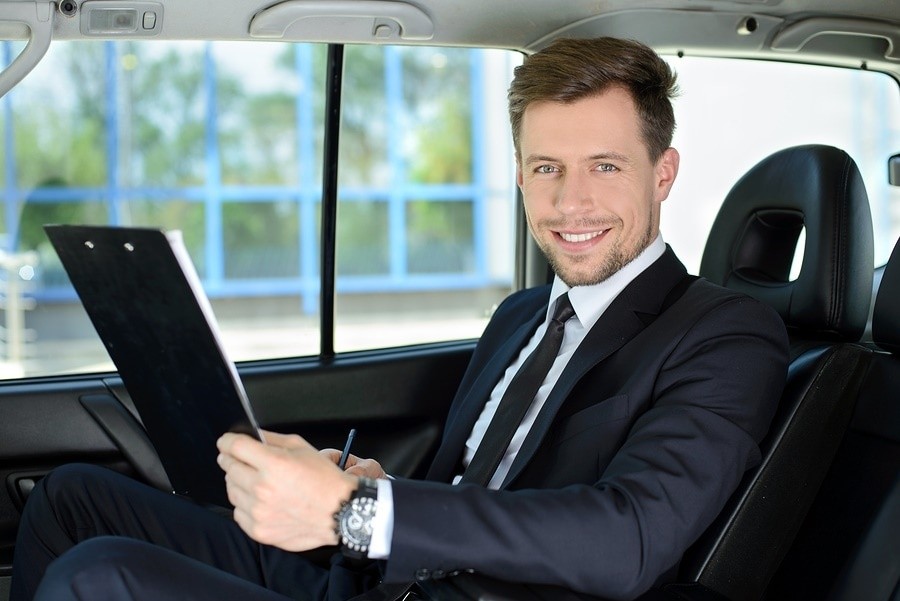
[338,428,356,472]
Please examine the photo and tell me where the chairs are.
[688,141,874,591]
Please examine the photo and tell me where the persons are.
[12,37,788,601]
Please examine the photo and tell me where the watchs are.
[331,477,378,564]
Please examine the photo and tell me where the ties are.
[457,292,576,489]
